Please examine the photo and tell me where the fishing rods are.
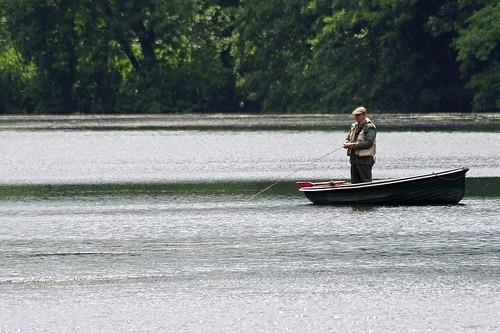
[246,146,345,201]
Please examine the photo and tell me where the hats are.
[352,107,367,114]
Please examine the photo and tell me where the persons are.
[343,107,377,183]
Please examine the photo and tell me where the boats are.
[295,166,472,207]
